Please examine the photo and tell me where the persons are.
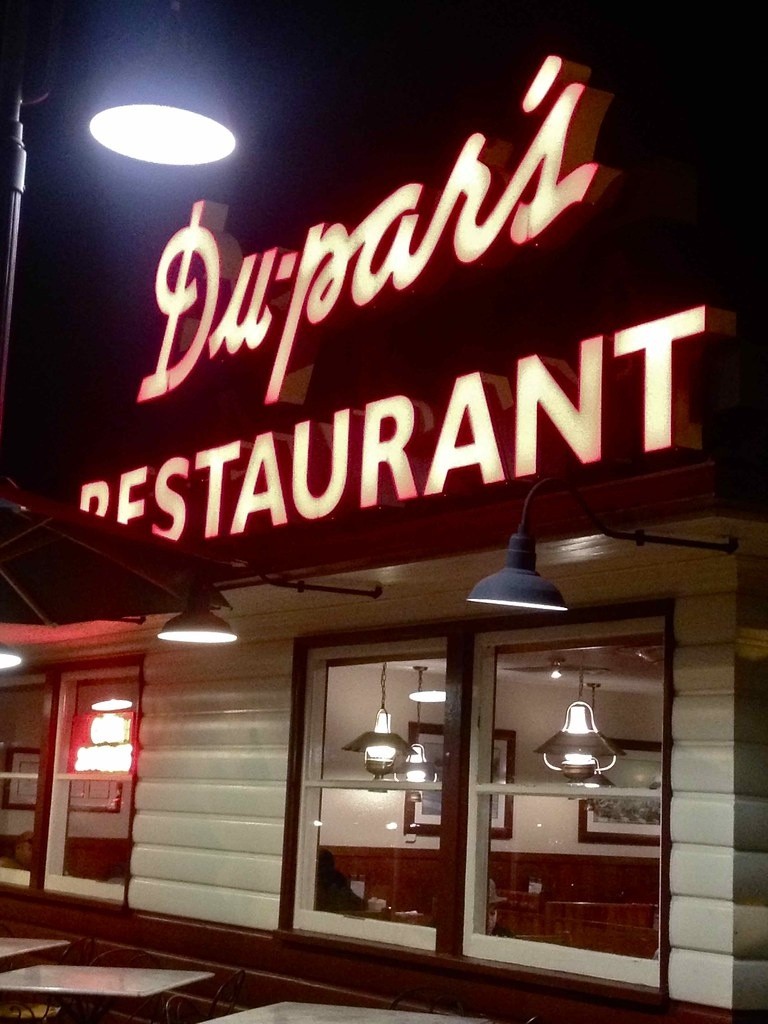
[317,845,368,910]
[0,831,38,871]
[485,880,516,937]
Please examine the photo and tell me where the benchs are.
[491,892,660,960]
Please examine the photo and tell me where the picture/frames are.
[403,721,516,840]
[0,746,40,811]
[577,737,663,846]
[68,781,125,813]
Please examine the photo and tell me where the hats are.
[488,878,507,903]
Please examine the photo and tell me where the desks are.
[199,1002,491,1024]
[0,965,216,1024]
[0,937,71,958]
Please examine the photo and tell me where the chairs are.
[387,987,465,1017]
[0,938,248,1024]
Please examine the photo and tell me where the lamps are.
[467,477,741,611]
[394,665,438,802]
[531,653,627,802]
[157,556,382,645]
[340,658,418,793]
